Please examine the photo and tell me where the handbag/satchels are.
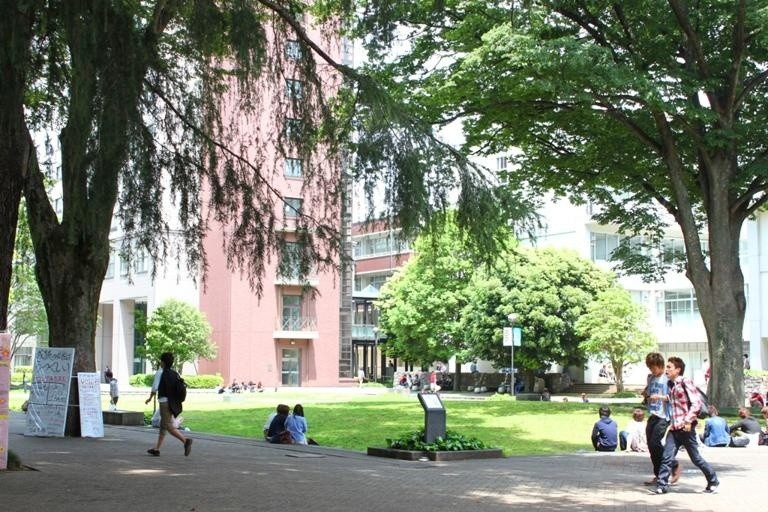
[729,436,750,447]
[682,378,708,418]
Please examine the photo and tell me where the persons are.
[644,357,721,495]
[744,354,749,368]
[145,352,192,457]
[358,366,364,384]
[591,392,768,458]
[703,359,710,384]
[640,352,685,487]
[218,378,264,394]
[263,404,319,445]
[581,392,590,402]
[471,359,479,379]
[399,370,452,394]
[105,366,119,411]
[501,371,526,393]
[542,387,550,401]
[388,360,394,367]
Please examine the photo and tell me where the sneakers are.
[703,480,719,493]
[645,463,681,495]
[147,447,161,456]
[184,438,193,456]
